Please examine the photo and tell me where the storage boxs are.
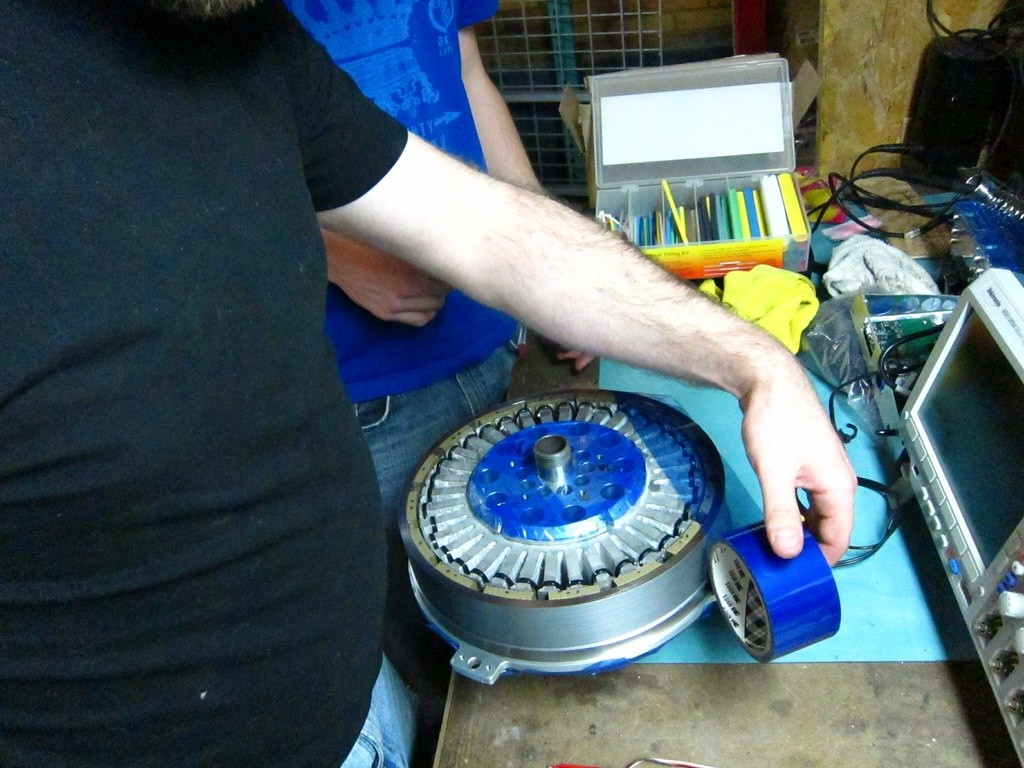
[585,53,810,283]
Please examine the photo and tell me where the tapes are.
[705,522,846,662]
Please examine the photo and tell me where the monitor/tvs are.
[915,308,1024,574]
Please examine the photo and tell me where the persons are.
[0,0,857,768]
[284,0,601,705]
